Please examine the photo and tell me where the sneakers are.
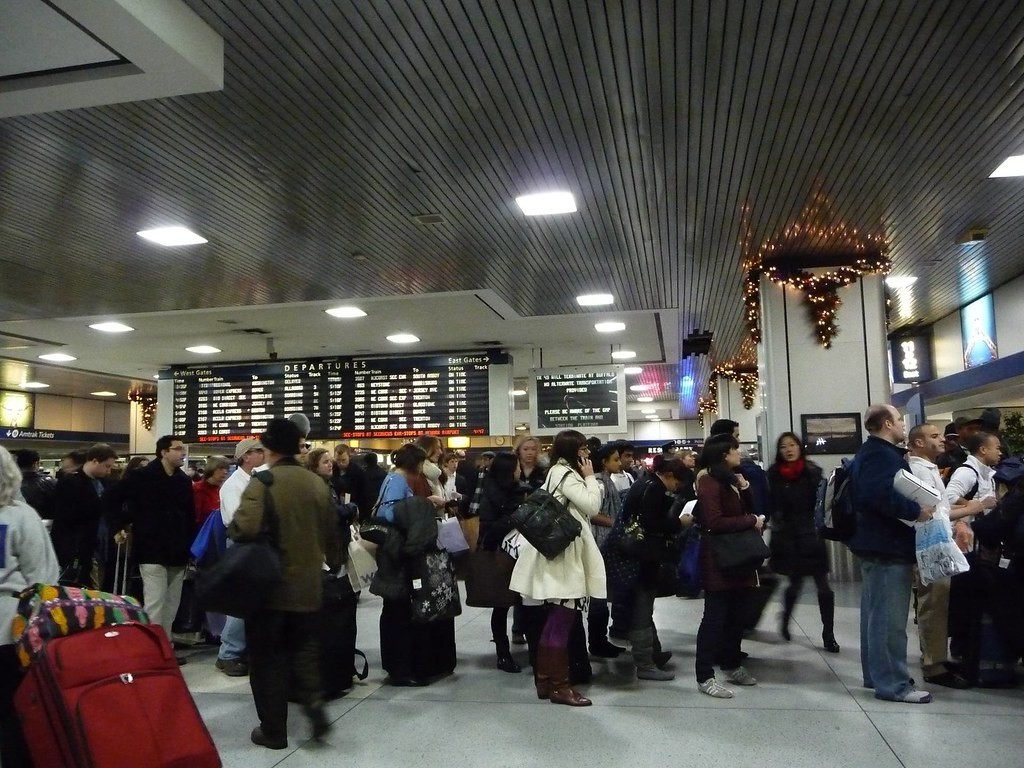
[867,662,971,703]
[698,666,757,698]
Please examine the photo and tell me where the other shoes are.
[176,646,288,749]
[389,674,429,687]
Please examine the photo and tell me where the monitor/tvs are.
[802,412,862,456]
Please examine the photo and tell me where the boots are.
[492,605,674,706]
[818,592,840,653]
[780,604,793,641]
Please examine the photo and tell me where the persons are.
[765,432,839,654]
[0,412,778,768]
[846,403,1024,704]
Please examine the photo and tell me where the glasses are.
[298,443,305,449]
[247,449,264,453]
[579,445,589,451]
[169,446,185,451]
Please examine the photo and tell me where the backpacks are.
[817,456,857,542]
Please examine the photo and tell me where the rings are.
[579,464,582,467]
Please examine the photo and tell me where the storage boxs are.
[890,468,941,528]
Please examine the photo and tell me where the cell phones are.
[576,455,583,466]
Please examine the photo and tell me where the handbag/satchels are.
[915,520,970,584]
[193,470,281,619]
[320,569,369,698]
[336,485,773,625]
[12,584,151,672]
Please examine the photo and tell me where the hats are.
[954,415,986,432]
[661,441,678,450]
[199,414,310,470]
[944,422,960,437]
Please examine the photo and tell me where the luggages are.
[12,620,223,768]
